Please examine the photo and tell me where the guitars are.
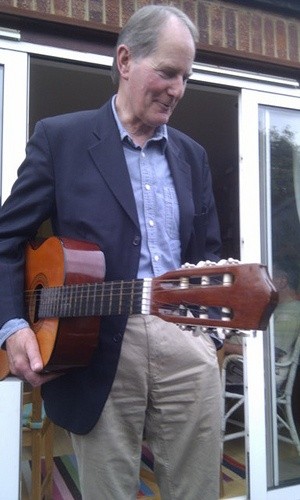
[0,238,280,384]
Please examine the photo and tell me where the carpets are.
[28,441,246,500]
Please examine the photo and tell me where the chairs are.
[222,331,300,444]
[19,378,55,500]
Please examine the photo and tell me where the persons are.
[0,3,231,500]
[208,254,300,392]
